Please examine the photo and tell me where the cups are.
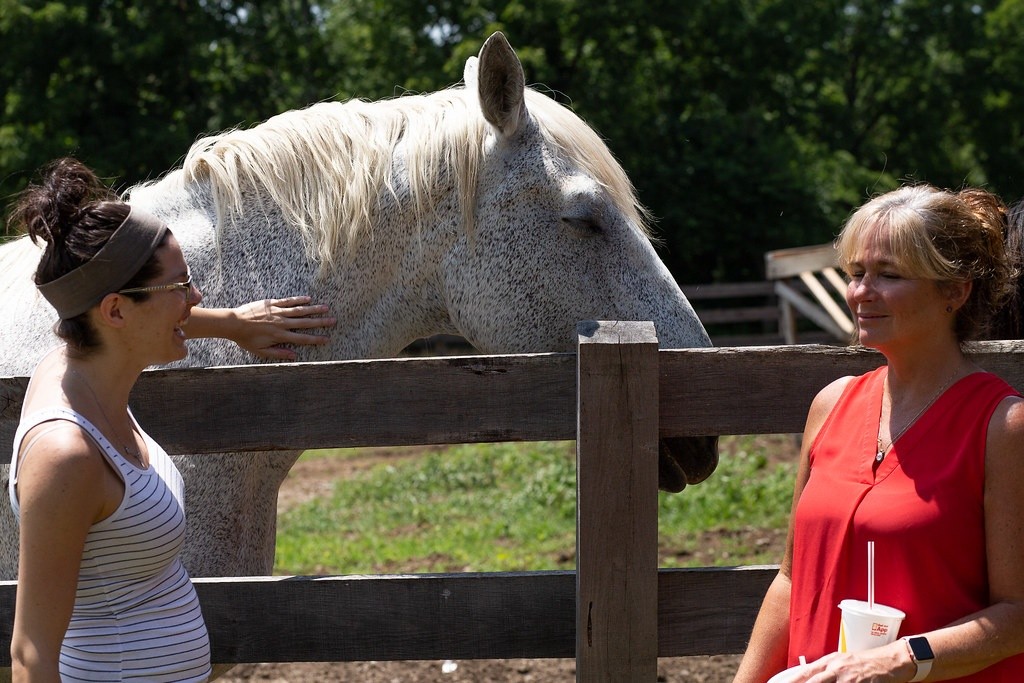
[837,600,906,652]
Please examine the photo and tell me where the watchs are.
[899,636,934,682]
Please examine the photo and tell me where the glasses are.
[119,265,195,297]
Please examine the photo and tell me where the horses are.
[0,29,720,683]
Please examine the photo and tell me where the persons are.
[721,186,1023,683]
[9,200,334,683]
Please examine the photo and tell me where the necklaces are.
[71,367,146,469]
[876,354,967,465]
